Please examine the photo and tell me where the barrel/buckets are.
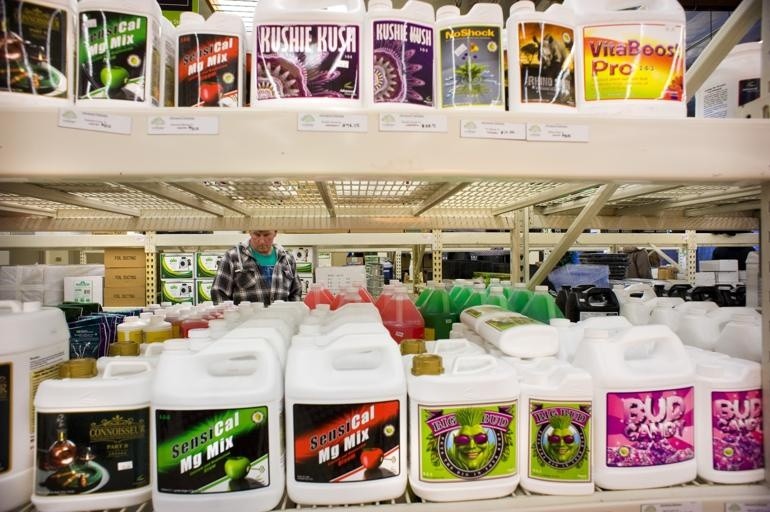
[285,334,408,505]
[0,298,71,512]
[450,279,465,299]
[407,354,520,501]
[612,284,658,309]
[366,1,436,110]
[714,310,761,362]
[174,11,247,110]
[375,279,425,345]
[458,284,486,312]
[550,315,633,340]
[486,278,501,294]
[304,280,336,309]
[574,326,697,490]
[436,2,504,112]
[620,297,686,326]
[521,285,565,324]
[506,0,575,114]
[697,358,763,484]
[654,283,747,307]
[447,322,473,337]
[576,1,686,117]
[677,307,761,346]
[151,339,286,512]
[648,301,719,326]
[555,285,596,316]
[161,15,174,108]
[501,281,512,297]
[62,299,390,338]
[566,288,620,322]
[250,1,366,109]
[509,283,533,312]
[245,34,252,106]
[336,279,375,308]
[421,282,458,338]
[0,0,76,108]
[400,338,489,372]
[477,315,560,358]
[76,0,161,108]
[454,281,474,308]
[31,356,150,512]
[415,280,436,307]
[520,369,594,494]
[459,304,536,333]
[485,286,509,308]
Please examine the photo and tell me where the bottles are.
[117,300,235,355]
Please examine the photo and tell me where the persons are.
[210,230,303,307]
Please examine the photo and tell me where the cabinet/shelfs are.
[0,85,768,511]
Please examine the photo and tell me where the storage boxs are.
[696,260,739,289]
[650,267,677,280]
[160,252,225,303]
[423,253,432,282]
[285,247,367,296]
[401,252,410,270]
[63,276,103,307]
[102,248,147,306]
